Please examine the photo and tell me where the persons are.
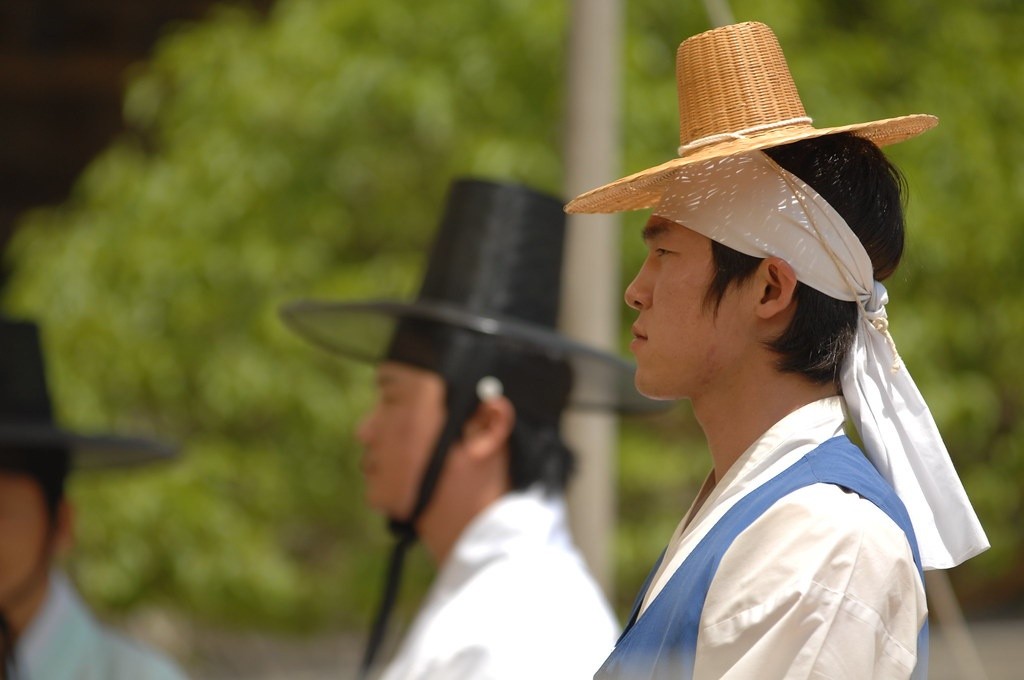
[562,24,990,680]
[275,174,671,680]
[0,320,189,678]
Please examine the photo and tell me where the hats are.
[562,21,940,215]
[0,322,178,473]
[275,174,684,416]
